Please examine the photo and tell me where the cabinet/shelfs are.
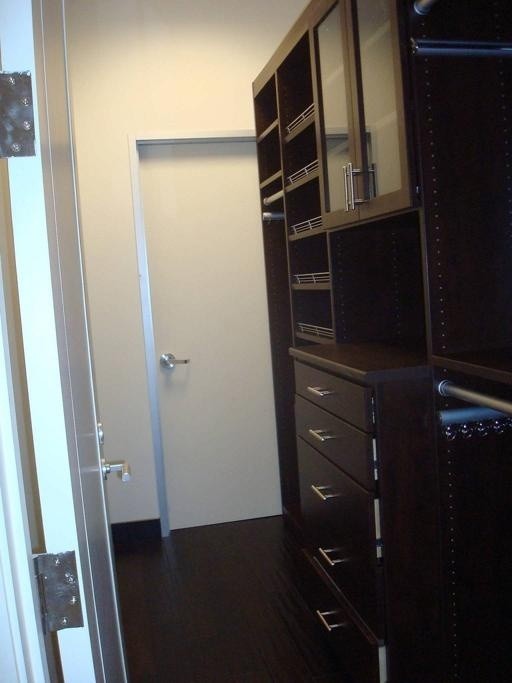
[251,0,512,683]
